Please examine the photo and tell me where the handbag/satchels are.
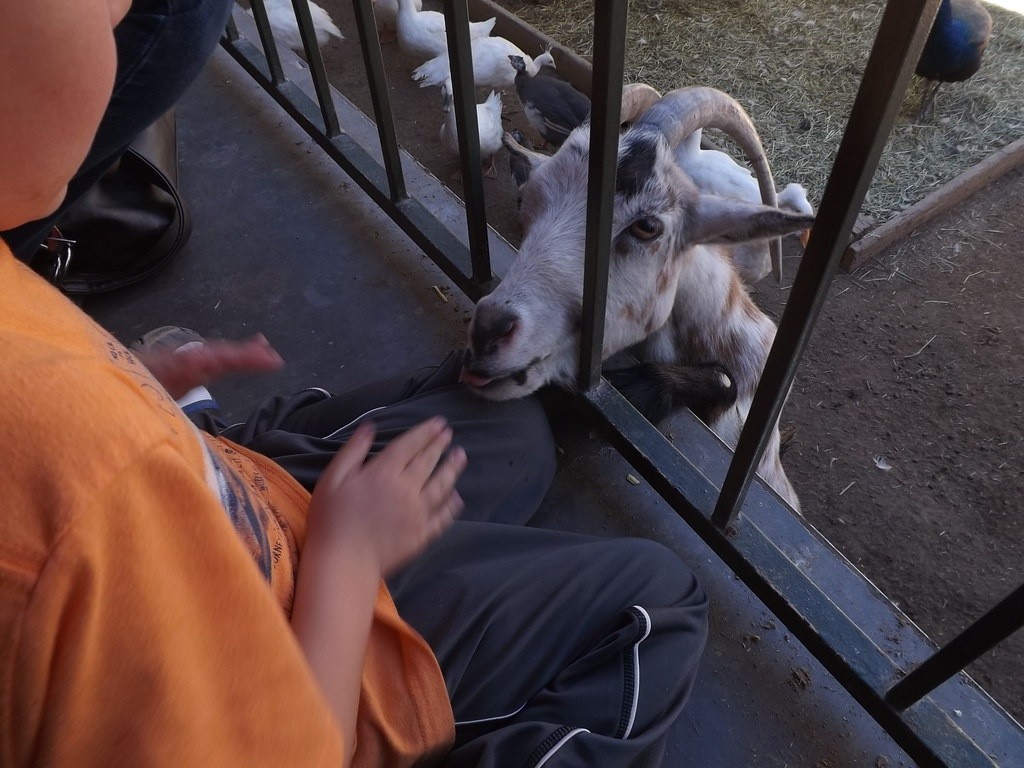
[0,103,193,297]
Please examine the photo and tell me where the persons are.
[0,0,707,768]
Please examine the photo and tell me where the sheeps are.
[457,80,816,521]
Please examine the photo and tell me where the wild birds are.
[898,0,993,121]
[242,0,592,213]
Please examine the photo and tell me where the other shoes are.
[127,326,223,417]
